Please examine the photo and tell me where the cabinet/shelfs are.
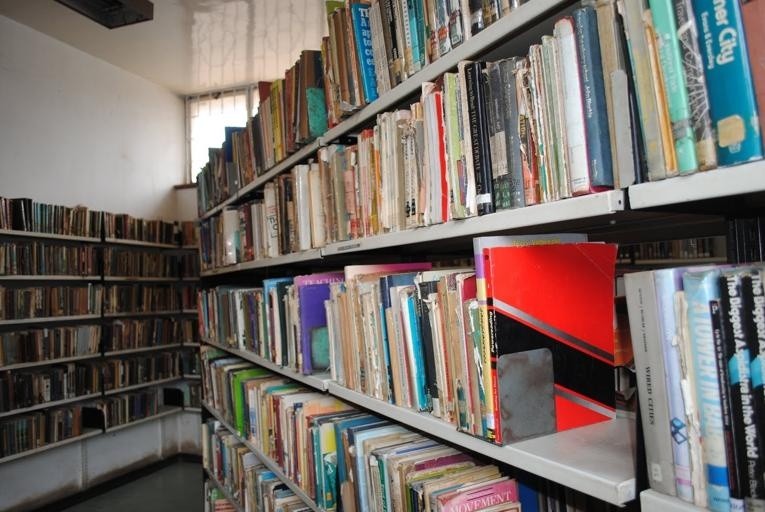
[199,1,765,512]
[181,245,201,462]
[0,229,181,512]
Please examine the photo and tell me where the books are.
[104,390,158,427]
[200,232,765,512]
[198,2,765,268]
[0,324,102,364]
[1,365,102,457]
[0,242,199,277]
[104,352,181,388]
[112,319,195,349]
[0,286,199,320]
[621,265,765,510]
[0,198,195,245]
